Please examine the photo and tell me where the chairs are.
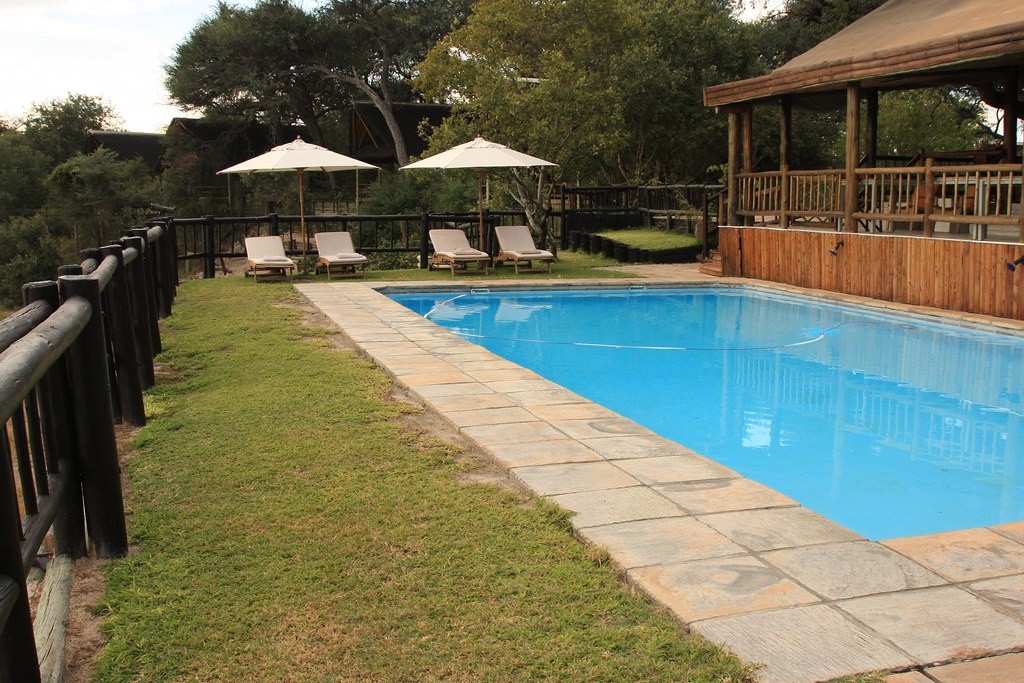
[314,232,367,280]
[954,185,988,238]
[244,236,294,283]
[493,226,553,275]
[894,184,938,231]
[429,229,491,277]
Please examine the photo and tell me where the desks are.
[834,177,883,232]
